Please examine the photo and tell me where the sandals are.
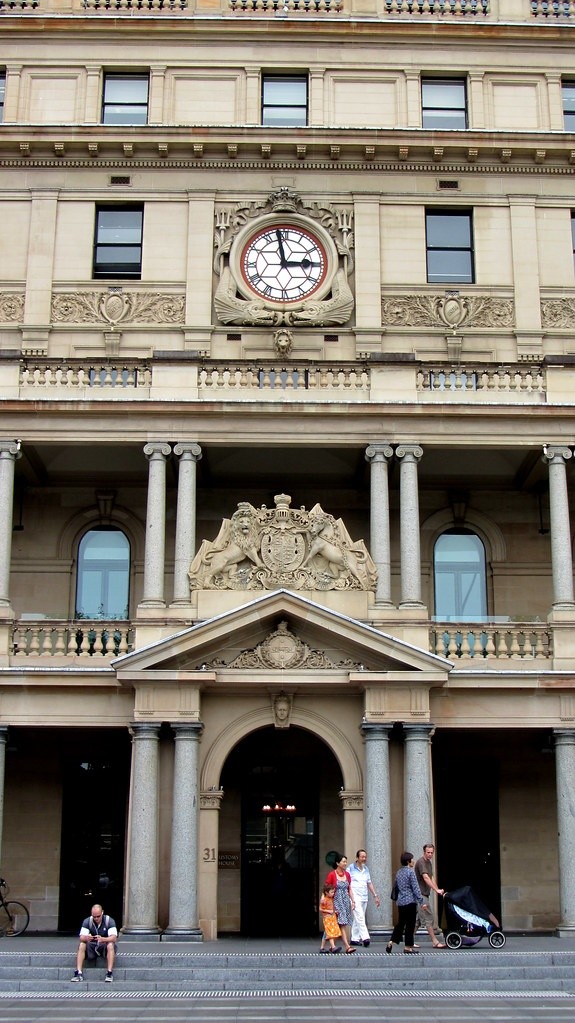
[329,947,332,952]
[346,947,357,953]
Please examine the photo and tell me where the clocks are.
[229,212,339,313]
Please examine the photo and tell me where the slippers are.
[413,943,420,948]
[433,942,447,948]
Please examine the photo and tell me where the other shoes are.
[386,944,393,952]
[404,948,419,953]
[350,940,362,946]
[363,939,370,947]
[320,948,329,953]
[332,946,342,952]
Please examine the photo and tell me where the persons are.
[70,904,117,981]
[346,849,380,947]
[413,843,446,949]
[325,855,356,954]
[386,853,428,953]
[319,884,342,953]
[275,695,289,725]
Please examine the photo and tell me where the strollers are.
[439,885,506,949]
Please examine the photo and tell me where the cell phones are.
[93,936,98,942]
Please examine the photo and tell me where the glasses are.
[93,912,103,918]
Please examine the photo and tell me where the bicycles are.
[0,878,30,937]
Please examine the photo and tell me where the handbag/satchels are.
[391,882,399,901]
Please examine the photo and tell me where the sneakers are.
[105,971,113,982]
[70,970,83,981]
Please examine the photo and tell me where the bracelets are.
[373,894,378,898]
